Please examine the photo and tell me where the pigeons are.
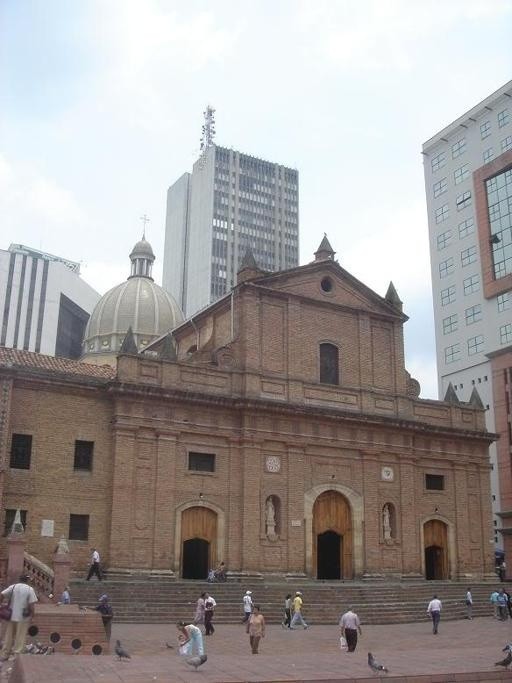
[186,654,207,671]
[166,641,174,649]
[22,639,51,656]
[494,643,512,669]
[55,602,62,606]
[81,608,88,614]
[368,653,388,677]
[115,640,131,661]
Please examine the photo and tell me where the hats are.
[98,595,109,603]
[295,591,302,596]
[246,590,252,595]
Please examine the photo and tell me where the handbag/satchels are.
[340,637,350,652]
[0,584,16,622]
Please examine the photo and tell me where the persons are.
[191,596,206,634]
[280,592,292,628]
[60,585,73,603]
[85,593,114,645]
[245,605,266,655]
[0,572,39,665]
[265,497,275,522]
[176,620,204,657]
[339,604,362,653]
[290,590,311,629]
[382,503,391,528]
[177,634,189,654]
[214,561,226,582]
[240,589,254,624]
[203,590,217,635]
[464,587,473,620]
[206,567,214,581]
[85,546,103,581]
[425,594,443,635]
[488,586,512,621]
